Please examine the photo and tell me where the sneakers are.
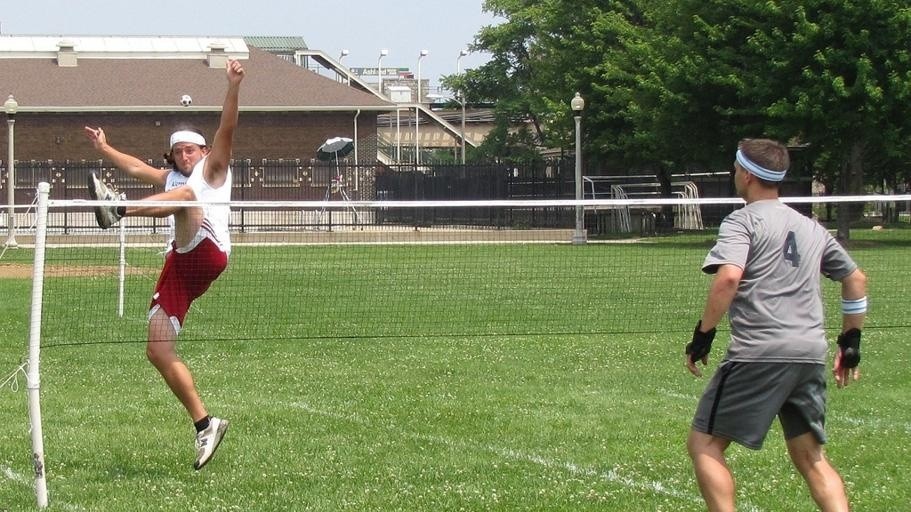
[193,416,230,470]
[88,168,126,230]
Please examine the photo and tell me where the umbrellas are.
[315,137,355,180]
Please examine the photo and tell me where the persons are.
[84,58,245,471]
[684,138,867,512]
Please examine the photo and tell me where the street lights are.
[571,93,586,238]
[3,94,16,246]
[334,48,469,164]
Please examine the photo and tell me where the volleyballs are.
[179,95,192,107]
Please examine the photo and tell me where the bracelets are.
[839,297,866,315]
